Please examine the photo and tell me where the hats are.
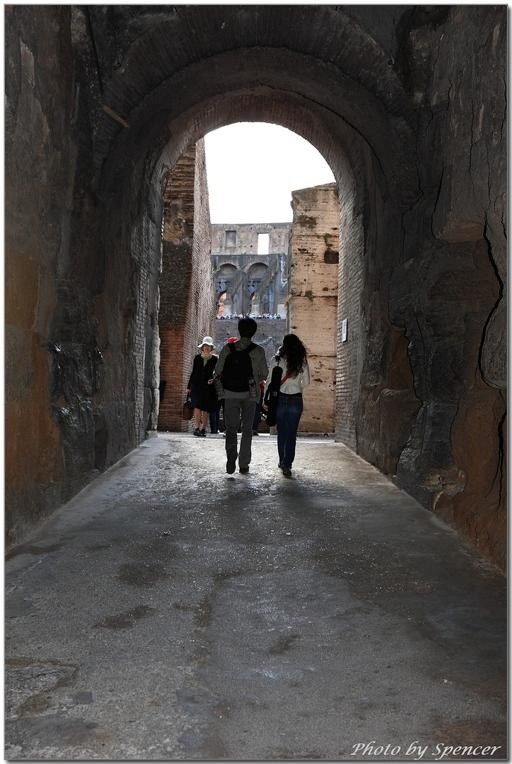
[197,335,216,351]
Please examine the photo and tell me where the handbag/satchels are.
[264,355,283,408]
[183,391,194,420]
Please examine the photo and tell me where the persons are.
[211,317,268,474]
[214,312,281,319]
[265,333,310,477]
[185,335,218,437]
[212,336,283,439]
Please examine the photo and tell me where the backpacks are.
[220,342,259,398]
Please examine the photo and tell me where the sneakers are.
[194,427,227,438]
[227,449,292,479]
